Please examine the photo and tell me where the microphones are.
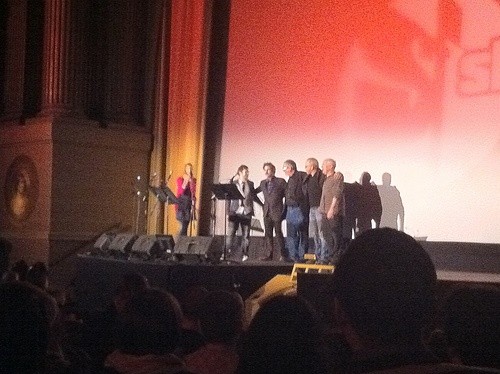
[188,174,191,182]
[233,173,238,178]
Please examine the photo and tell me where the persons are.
[174,163,197,236]
[237,285,500,374]
[255,163,288,262]
[1,238,208,374]
[222,165,264,262]
[319,159,344,265]
[184,289,245,373]
[283,159,309,262]
[302,158,344,264]
[101,287,187,374]
[333,227,443,374]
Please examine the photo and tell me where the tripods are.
[208,183,245,264]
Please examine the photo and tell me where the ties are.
[241,182,245,206]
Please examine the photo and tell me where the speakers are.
[93,233,113,256]
[173,235,224,264]
[131,235,164,260]
[108,233,139,258]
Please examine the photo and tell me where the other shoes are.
[259,257,272,261]
[242,254,248,261]
[220,254,227,260]
[279,256,286,262]
[172,254,178,262]
